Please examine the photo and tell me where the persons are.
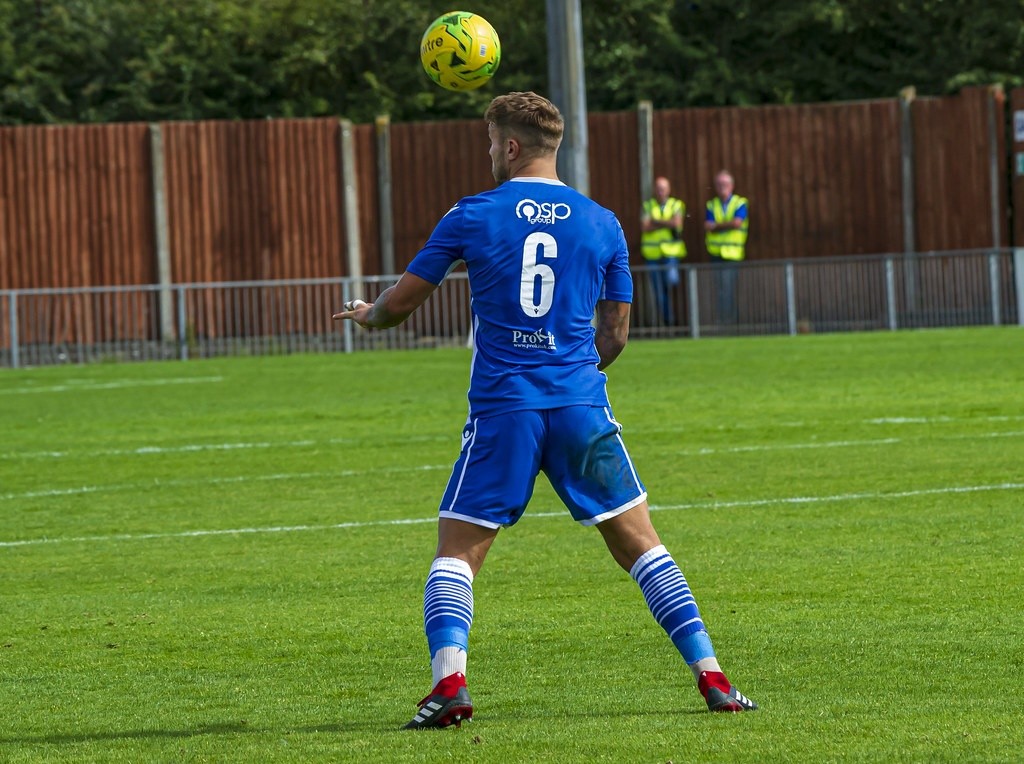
[640,176,687,338]
[703,170,749,325]
[334,91,755,731]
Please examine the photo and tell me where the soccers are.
[420,10,501,92]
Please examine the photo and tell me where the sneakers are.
[706,685,759,712]
[402,671,473,730]
